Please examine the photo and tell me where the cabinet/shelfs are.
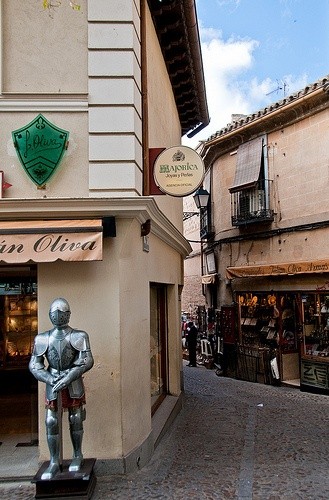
[238,292,329,381]
[0,294,38,395]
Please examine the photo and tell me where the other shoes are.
[186,363,191,366]
[189,365,196,367]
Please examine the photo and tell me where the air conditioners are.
[238,189,264,217]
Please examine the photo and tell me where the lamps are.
[193,185,210,214]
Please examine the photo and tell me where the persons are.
[29,298,93,480]
[185,321,198,367]
